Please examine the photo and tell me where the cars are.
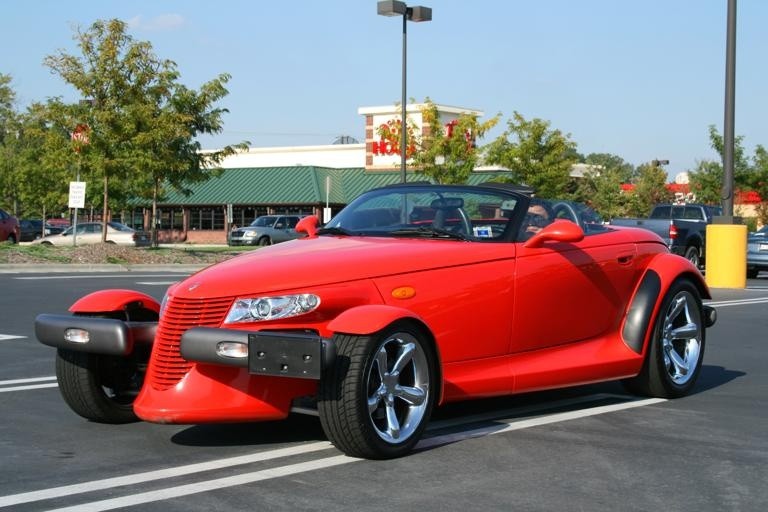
[0,209,153,249]
[747,225,768,279]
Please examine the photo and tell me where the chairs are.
[551,200,587,233]
[432,204,473,237]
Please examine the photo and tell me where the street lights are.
[652,160,670,168]
[377,1,434,222]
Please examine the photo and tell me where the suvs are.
[226,212,312,249]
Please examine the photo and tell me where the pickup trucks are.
[609,202,722,270]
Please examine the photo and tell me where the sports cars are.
[32,179,720,463]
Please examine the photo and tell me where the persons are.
[522,199,555,236]
